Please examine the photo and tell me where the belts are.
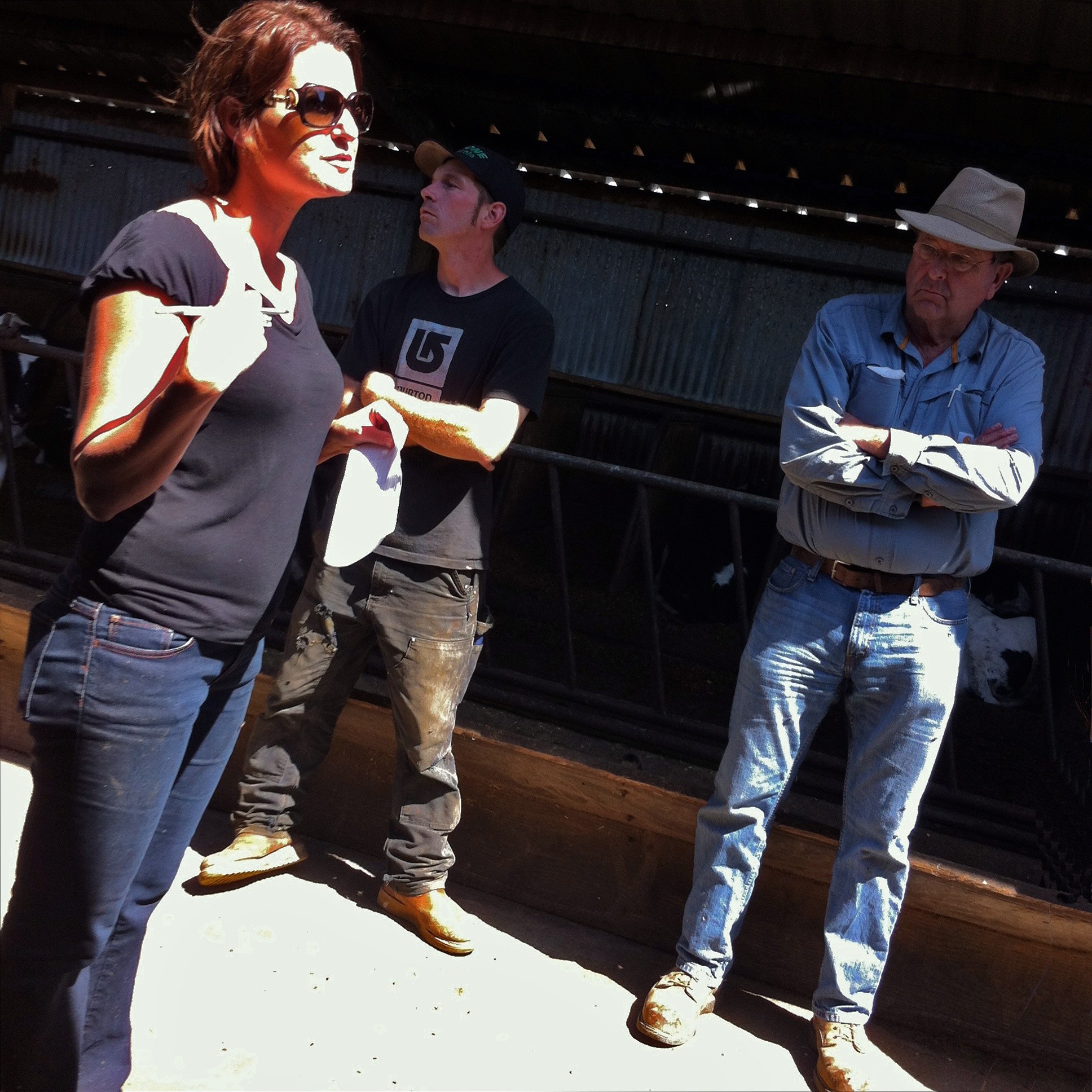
[778,537,965,596]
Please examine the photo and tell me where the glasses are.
[261,82,373,135]
[913,242,1006,272]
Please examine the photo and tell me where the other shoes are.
[635,969,718,1045]
[804,1015,871,1092]
[377,871,477,955]
[199,824,305,888]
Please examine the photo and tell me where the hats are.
[414,140,524,220]
[895,167,1039,282]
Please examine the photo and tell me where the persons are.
[195,131,561,959]
[632,162,1044,1091]
[0,2,405,1092]
